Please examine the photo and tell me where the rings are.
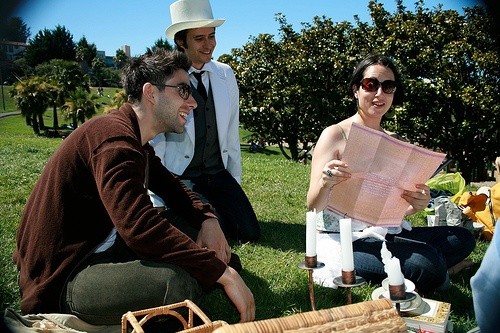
[327,169,333,177]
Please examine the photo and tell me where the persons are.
[307,54,476,296]
[15,44,256,324]
[144,0,259,271]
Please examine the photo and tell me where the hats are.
[164,0,225,39]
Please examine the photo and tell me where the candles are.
[339,213,354,272]
[305,207,317,257]
[386,255,404,286]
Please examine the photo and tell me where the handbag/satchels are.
[4,308,122,333]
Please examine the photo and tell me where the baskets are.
[122,299,408,333]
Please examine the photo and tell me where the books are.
[374,298,451,333]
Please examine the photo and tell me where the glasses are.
[356,78,397,94]
[151,83,193,100]
